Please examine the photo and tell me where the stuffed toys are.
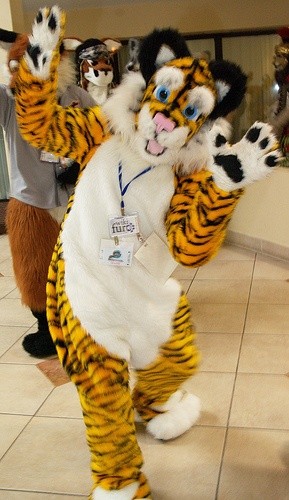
[63,37,121,104]
[16,5,282,500]
[0,29,97,358]
